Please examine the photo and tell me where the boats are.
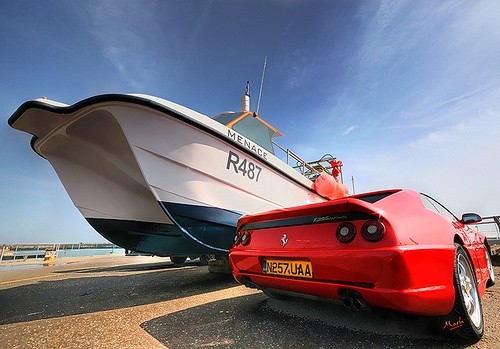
[7,56,351,275]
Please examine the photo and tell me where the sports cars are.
[228,189,495,341]
